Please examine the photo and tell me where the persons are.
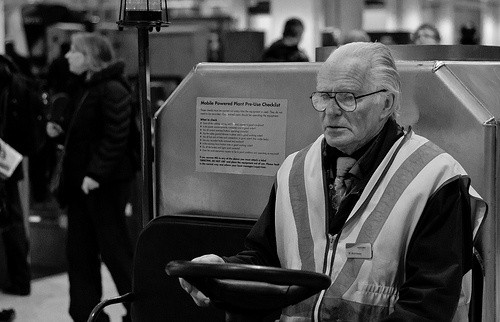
[261,19,309,61]
[178,40,488,322]
[0,0,147,322]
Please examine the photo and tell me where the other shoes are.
[0,309,15,322]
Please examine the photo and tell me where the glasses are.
[414,35,434,40]
[310,89,388,112]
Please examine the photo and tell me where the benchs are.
[86,215,487,322]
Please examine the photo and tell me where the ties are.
[331,156,355,216]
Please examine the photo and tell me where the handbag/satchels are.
[48,151,67,210]
